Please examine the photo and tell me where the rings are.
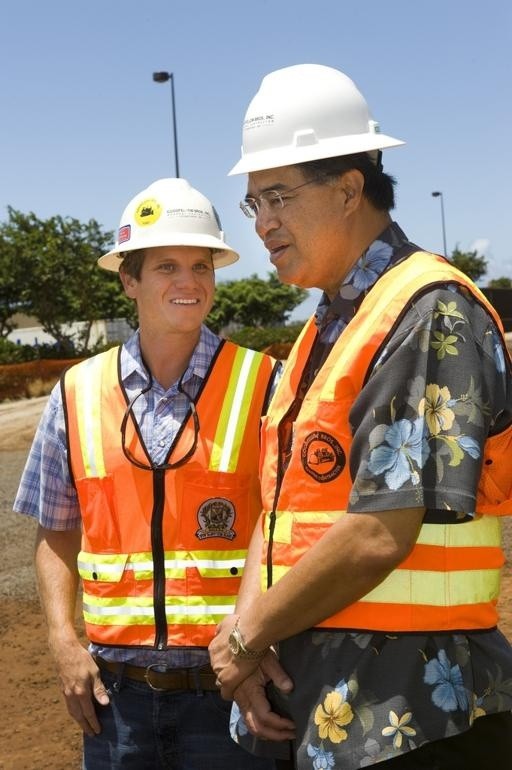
[214,677,224,690]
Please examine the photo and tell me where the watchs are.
[227,617,269,660]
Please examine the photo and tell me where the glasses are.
[121,431,199,470]
[241,184,315,219]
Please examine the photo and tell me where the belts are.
[93,659,217,692]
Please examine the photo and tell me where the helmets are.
[227,64,406,177]
[96,177,239,272]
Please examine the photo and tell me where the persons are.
[12,177,291,764]
[193,60,511,764]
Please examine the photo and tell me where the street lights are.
[154,71,180,179]
[431,192,447,260]
